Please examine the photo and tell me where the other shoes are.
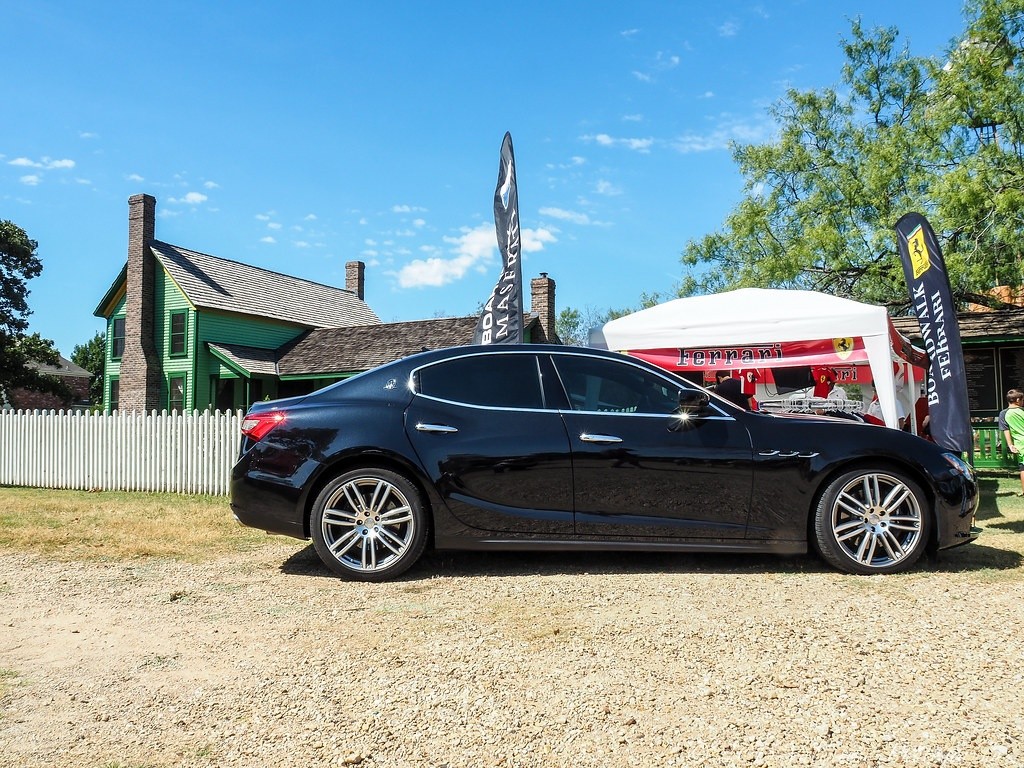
[1017,492,1024,498]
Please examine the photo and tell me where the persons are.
[998,388,1024,497]
[714,370,753,412]
[867,378,905,429]
[805,368,847,400]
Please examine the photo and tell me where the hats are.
[715,369,728,384]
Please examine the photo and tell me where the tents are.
[602,287,929,437]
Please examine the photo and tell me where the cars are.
[226,342,983,584]
